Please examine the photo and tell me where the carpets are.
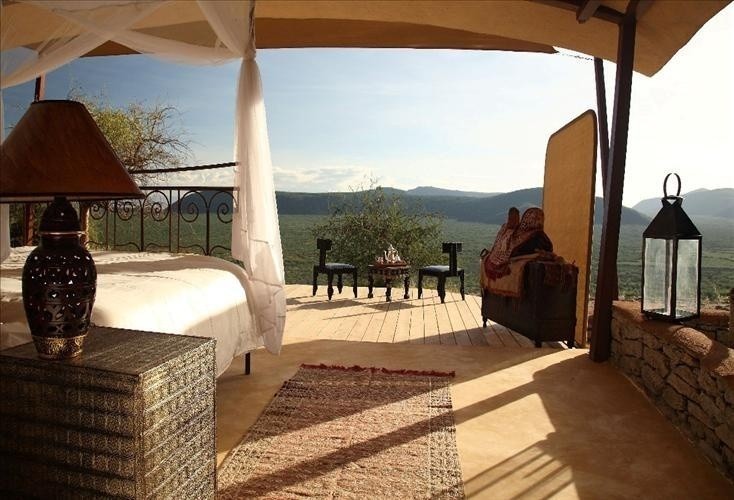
[204,364,464,500]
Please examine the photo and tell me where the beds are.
[1,0,285,380]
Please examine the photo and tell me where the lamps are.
[640,171,703,324]
[0,100,144,359]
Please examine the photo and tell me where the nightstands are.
[1,323,218,500]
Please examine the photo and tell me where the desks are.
[368,264,411,302]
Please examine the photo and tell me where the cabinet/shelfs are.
[481,249,579,348]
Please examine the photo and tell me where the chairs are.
[313,238,358,299]
[417,242,464,303]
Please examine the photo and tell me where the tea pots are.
[384,243,398,263]
